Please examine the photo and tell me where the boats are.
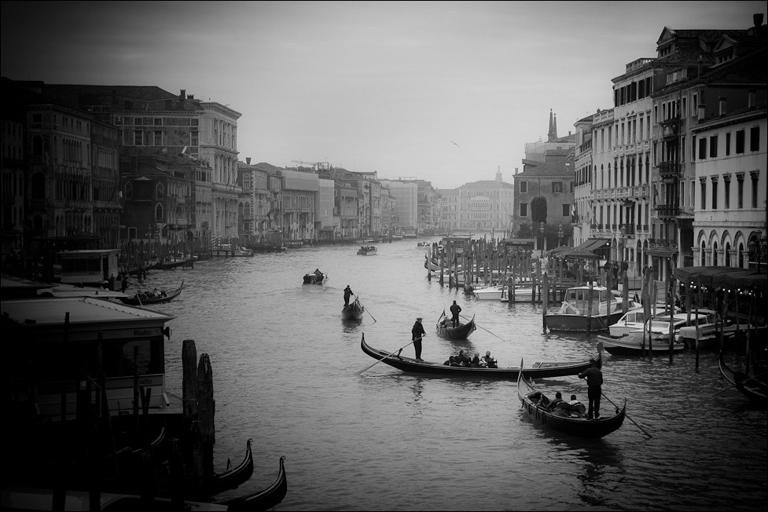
[360,332,602,379]
[517,358,627,439]
[718,348,766,401]
[343,296,365,320]
[1,298,286,511]
[304,273,328,283]
[357,244,380,258]
[114,279,185,304]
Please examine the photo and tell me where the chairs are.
[554,401,586,414]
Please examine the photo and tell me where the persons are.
[579,362,603,419]
[412,318,426,361]
[450,300,462,328]
[448,349,499,368]
[440,317,451,328]
[344,285,353,306]
[121,276,129,293]
[536,392,586,418]
